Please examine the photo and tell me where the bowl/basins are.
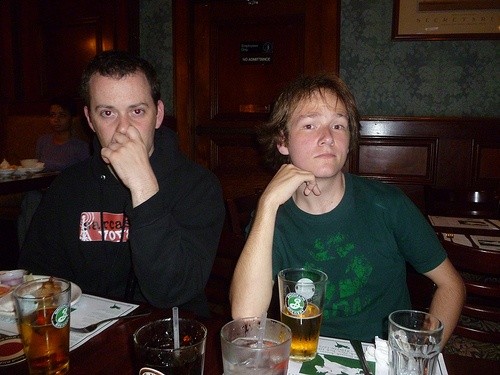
[0,269,24,287]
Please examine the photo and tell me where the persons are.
[19,48,223,317]
[229,75,465,354]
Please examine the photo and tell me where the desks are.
[0,171,60,196]
[0,306,500,375]
[405,208,500,343]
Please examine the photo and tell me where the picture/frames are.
[390,0,500,41]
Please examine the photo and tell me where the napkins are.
[374,335,389,375]
[428,214,500,251]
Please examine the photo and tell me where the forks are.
[446,232,454,244]
[69,309,149,333]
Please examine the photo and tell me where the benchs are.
[0,115,92,221]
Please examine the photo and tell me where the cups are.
[278,268,328,362]
[220,317,292,375]
[133,317,208,375]
[13,278,71,375]
[387,310,445,375]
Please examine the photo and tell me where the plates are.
[0,168,45,175]
[0,336,27,366]
[0,275,82,316]
